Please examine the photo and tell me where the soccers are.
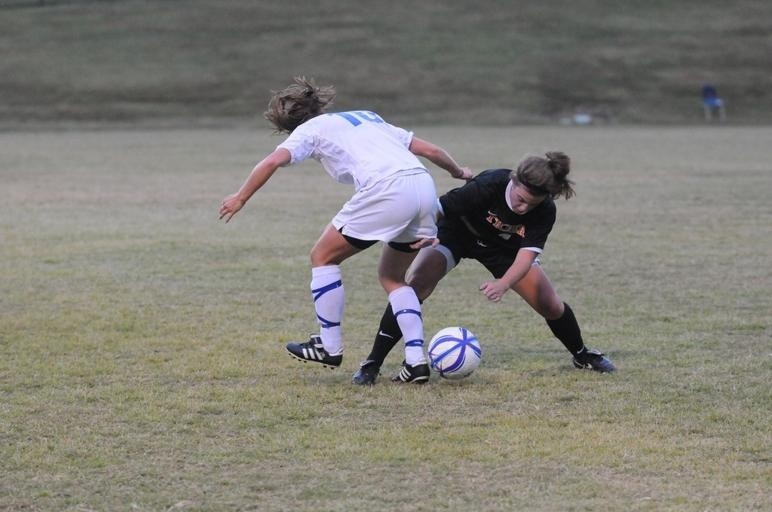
[427,325,483,380]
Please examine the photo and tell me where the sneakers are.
[391,359,430,385]
[572,349,617,374]
[352,359,380,385]
[285,335,343,367]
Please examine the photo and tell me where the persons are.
[351,151,617,382]
[216,73,476,383]
